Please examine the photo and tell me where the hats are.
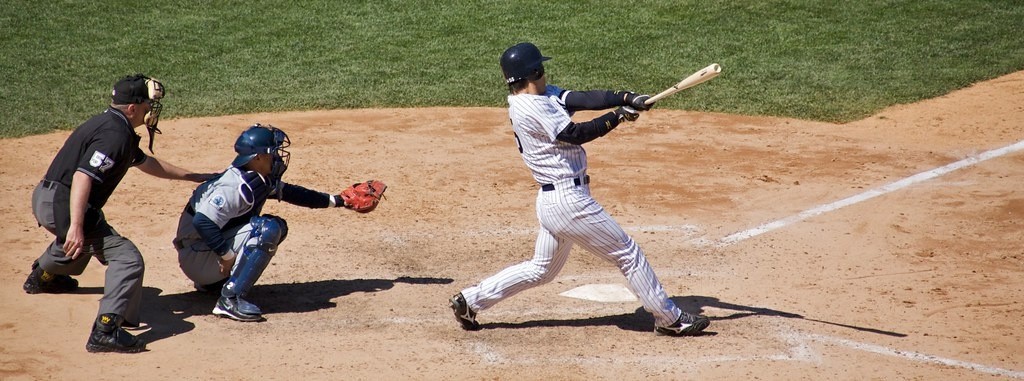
[111,73,148,105]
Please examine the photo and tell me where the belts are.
[41,180,58,189]
[174,239,185,251]
[542,175,590,192]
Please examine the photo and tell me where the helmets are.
[231,123,285,167]
[500,42,552,84]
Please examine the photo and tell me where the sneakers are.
[211,297,262,322]
[23,263,78,294]
[653,310,711,337]
[85,314,147,353]
[448,293,481,330]
[195,278,230,291]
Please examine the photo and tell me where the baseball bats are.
[644,63,723,105]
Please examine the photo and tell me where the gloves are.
[613,106,640,124]
[624,92,656,111]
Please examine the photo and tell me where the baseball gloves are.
[340,180,388,212]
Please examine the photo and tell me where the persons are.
[173,123,388,322]
[23,73,219,354]
[449,42,710,335]
